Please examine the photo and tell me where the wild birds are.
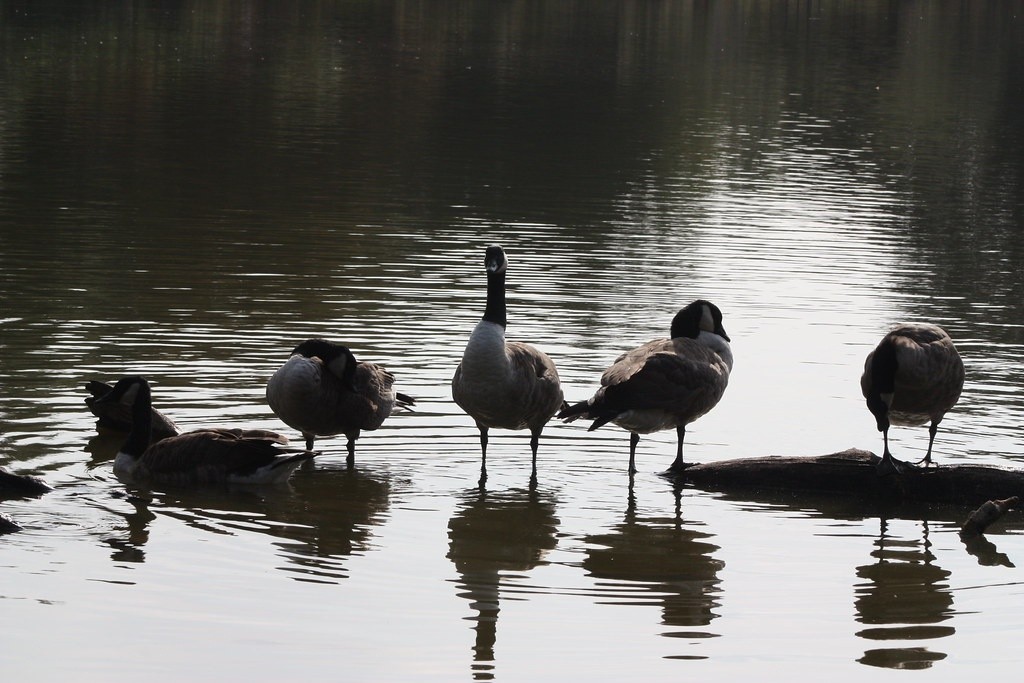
[85,374,322,494]
[556,299,732,475]
[452,245,564,480]
[860,322,965,480]
[265,339,416,457]
